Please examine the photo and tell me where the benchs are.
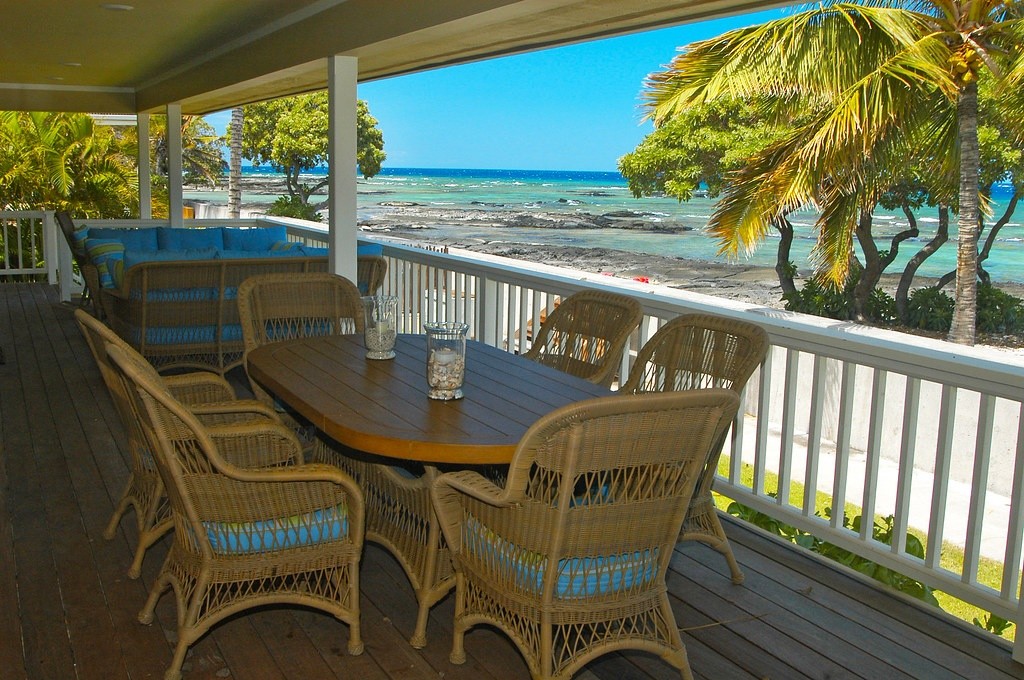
[96,253,387,379]
[79,257,105,322]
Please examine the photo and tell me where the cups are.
[424,322,468,402]
[361,295,397,360]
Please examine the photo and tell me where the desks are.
[244,329,612,648]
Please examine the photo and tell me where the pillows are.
[296,244,383,292]
[222,225,287,250]
[156,227,224,249]
[269,240,304,251]
[122,245,217,302]
[70,223,90,250]
[87,227,159,252]
[83,237,126,288]
[214,249,304,299]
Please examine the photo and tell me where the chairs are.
[73,307,303,580]
[54,209,91,307]
[517,288,642,388]
[607,311,771,583]
[428,388,739,678]
[101,338,365,680]
[237,272,366,455]
